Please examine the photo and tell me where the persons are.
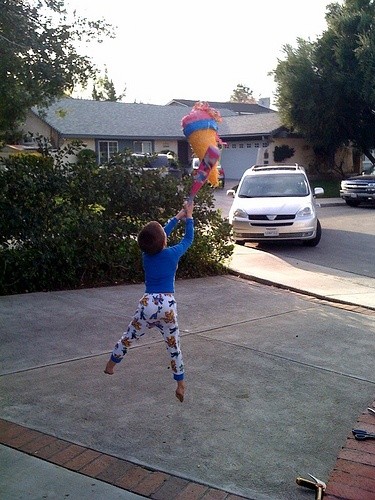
[104,200,194,401]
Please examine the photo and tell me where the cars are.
[340,163,375,210]
[190,156,226,190]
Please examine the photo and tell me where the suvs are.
[226,162,324,247]
[119,151,182,181]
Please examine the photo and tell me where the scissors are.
[352,429,375,440]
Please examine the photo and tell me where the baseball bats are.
[182,145,221,220]
